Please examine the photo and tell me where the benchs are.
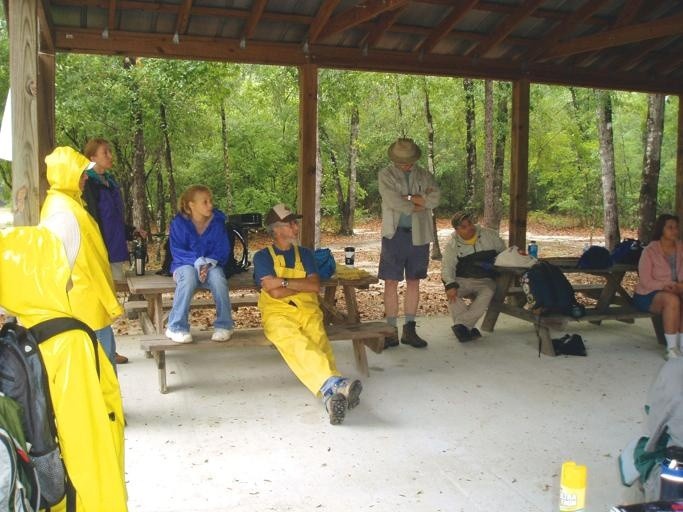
[464,283,608,299]
[538,307,662,321]
[139,321,397,352]
[124,294,338,320]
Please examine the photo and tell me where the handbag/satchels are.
[314,248,335,277]
[579,237,644,270]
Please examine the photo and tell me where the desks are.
[481,257,667,356]
[126,264,379,394]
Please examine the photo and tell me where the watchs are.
[406,193,414,202]
[281,278,289,288]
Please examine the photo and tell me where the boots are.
[401,321,427,347]
[384,327,399,348]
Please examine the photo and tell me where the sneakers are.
[452,324,481,341]
[326,378,362,424]
[166,328,192,344]
[664,346,681,360]
[211,327,233,341]
[115,353,128,363]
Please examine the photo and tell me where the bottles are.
[528,241,537,258]
[135,237,145,276]
[345,247,355,269]
[559,462,587,512]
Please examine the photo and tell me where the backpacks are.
[519,261,578,315]
[224,222,251,279]
[0,317,86,512]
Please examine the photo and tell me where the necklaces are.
[96,172,107,177]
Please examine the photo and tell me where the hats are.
[264,203,303,225]
[387,137,422,163]
[452,211,469,226]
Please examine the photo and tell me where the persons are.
[165,186,236,344]
[374,138,445,351]
[35,147,122,381]
[441,213,521,343]
[619,352,681,511]
[252,204,363,426]
[77,137,135,303]
[0,225,130,511]
[630,215,682,360]
